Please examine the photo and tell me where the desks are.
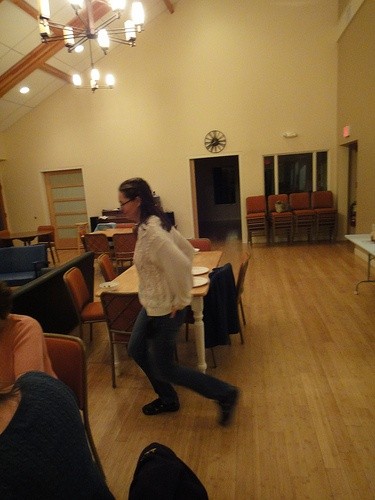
[81,228,133,237]
[344,234,375,294]
[96,251,223,376]
[0,231,53,246]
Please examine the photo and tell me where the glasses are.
[120,199,134,208]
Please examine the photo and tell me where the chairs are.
[0,223,252,500]
[246,191,337,247]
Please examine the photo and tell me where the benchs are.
[0,244,49,287]
[9,251,95,336]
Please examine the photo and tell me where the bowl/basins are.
[193,248,200,254]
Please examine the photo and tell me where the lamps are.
[37,0,145,93]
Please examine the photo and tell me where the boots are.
[204,378,238,425]
[142,383,179,415]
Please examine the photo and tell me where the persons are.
[0,369,116,500]
[118,177,239,427]
[0,282,58,394]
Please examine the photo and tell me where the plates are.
[192,277,209,287]
[192,266,210,275]
[98,282,118,289]
